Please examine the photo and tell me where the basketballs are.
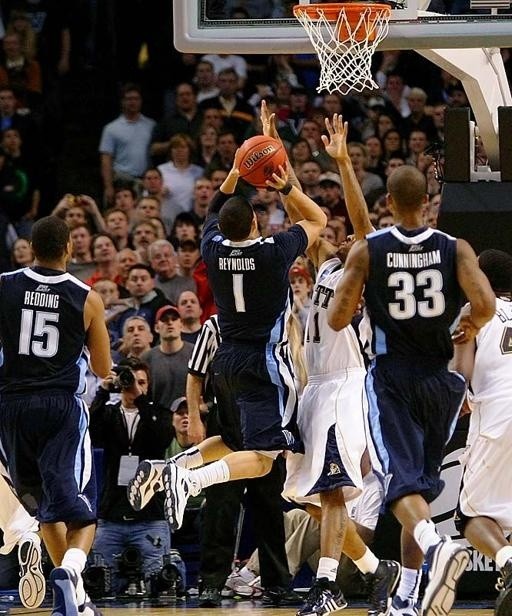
[236,134,286,188]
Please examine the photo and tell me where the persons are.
[0,0,512,616]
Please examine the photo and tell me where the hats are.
[155,305,180,319]
[170,397,188,411]
[287,266,312,286]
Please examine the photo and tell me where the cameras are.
[68,196,81,204]
[108,367,134,392]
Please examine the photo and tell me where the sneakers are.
[127,458,165,512]
[50,565,79,616]
[17,532,47,609]
[80,592,101,615]
[293,579,348,615]
[423,537,473,615]
[162,463,200,532]
[359,559,400,615]
[211,567,264,598]
[494,558,512,616]
[384,596,419,616]
